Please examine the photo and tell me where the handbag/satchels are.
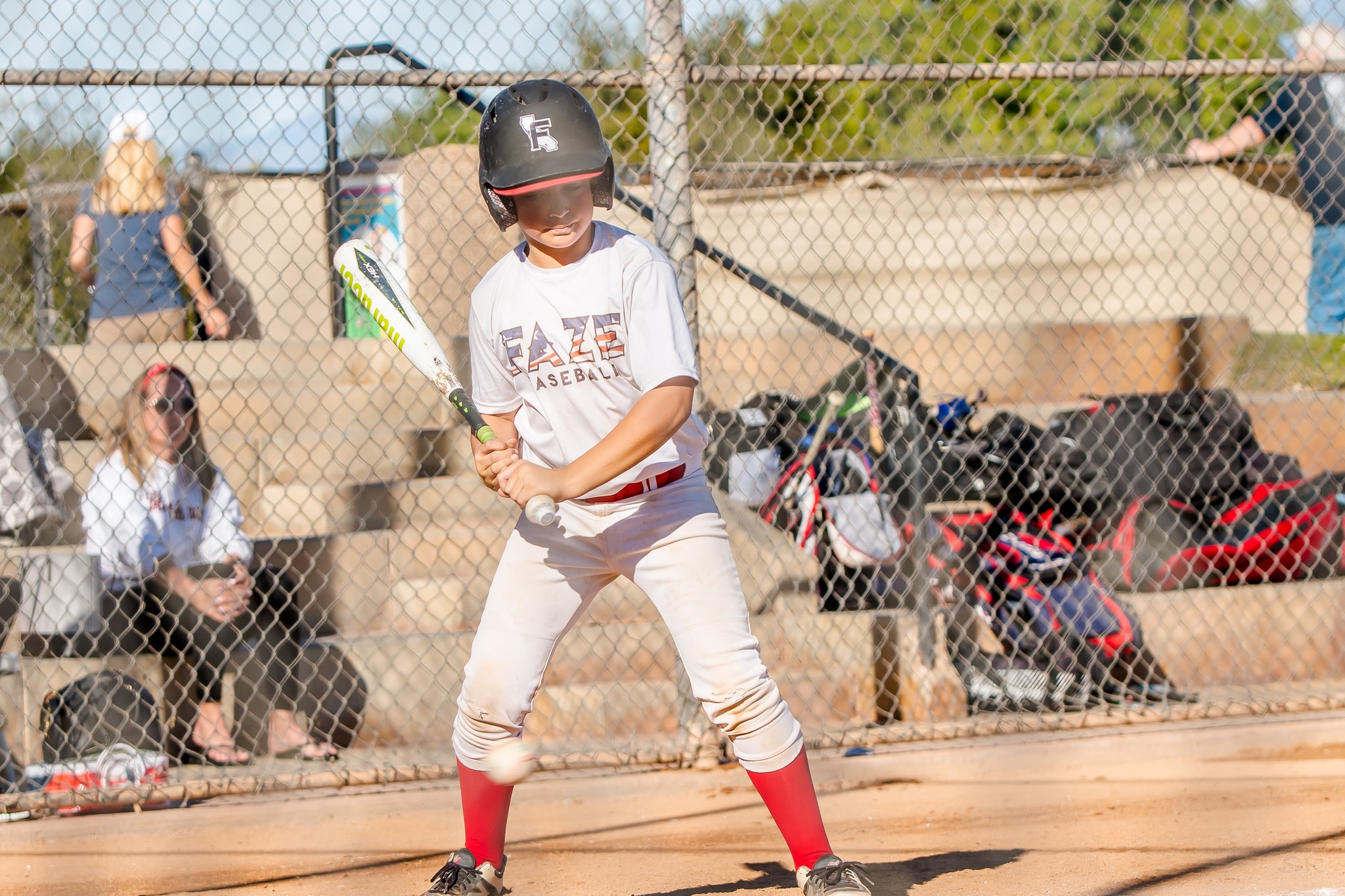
[39,669,165,761]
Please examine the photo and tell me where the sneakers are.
[796,855,876,896]
[420,848,508,896]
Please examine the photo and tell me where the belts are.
[581,463,686,505]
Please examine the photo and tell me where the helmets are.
[477,79,615,232]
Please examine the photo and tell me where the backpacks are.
[708,368,1345,708]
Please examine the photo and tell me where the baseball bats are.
[334,239,556,529]
[861,328,885,455]
[785,390,847,510]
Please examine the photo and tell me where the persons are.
[0,374,63,544]
[69,111,232,342]
[1185,24,1345,334]
[79,365,340,765]
[424,79,875,896]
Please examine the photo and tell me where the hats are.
[107,111,157,142]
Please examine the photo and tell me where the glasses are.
[145,397,198,416]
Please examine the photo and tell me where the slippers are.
[276,743,340,763]
[188,740,253,767]
